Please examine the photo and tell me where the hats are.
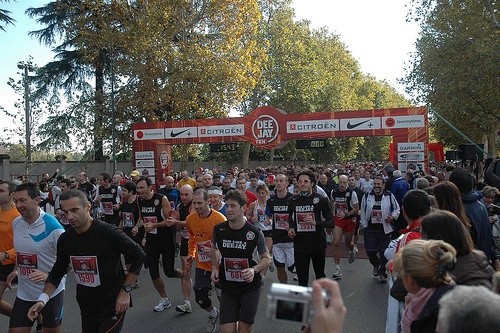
[131,170,140,176]
[385,166,395,174]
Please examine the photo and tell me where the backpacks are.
[48,185,58,206]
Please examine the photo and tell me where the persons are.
[167,184,215,313]
[246,185,275,285]
[303,278,346,333]
[361,177,400,283]
[287,171,335,287]
[172,162,395,219]
[27,189,146,333]
[131,176,193,312]
[435,285,500,333]
[385,154,500,279]
[331,175,360,280]
[7,183,66,333]
[119,182,145,289]
[392,239,456,333]
[185,188,228,333]
[264,174,298,284]
[12,168,142,227]
[0,179,43,333]
[156,176,182,257]
[420,210,500,296]
[210,189,271,333]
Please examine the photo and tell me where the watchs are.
[121,284,134,293]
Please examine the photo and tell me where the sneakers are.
[373,264,379,278]
[175,302,193,313]
[379,272,388,283]
[349,249,355,264]
[206,307,220,333]
[153,298,171,312]
[332,267,343,280]
[353,244,358,255]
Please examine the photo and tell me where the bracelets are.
[37,292,49,305]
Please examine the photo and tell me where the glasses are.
[298,179,312,183]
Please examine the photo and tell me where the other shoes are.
[293,274,299,280]
[135,280,140,289]
[269,261,275,273]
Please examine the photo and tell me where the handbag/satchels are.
[390,277,407,302]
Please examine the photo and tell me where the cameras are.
[266,282,328,326]
